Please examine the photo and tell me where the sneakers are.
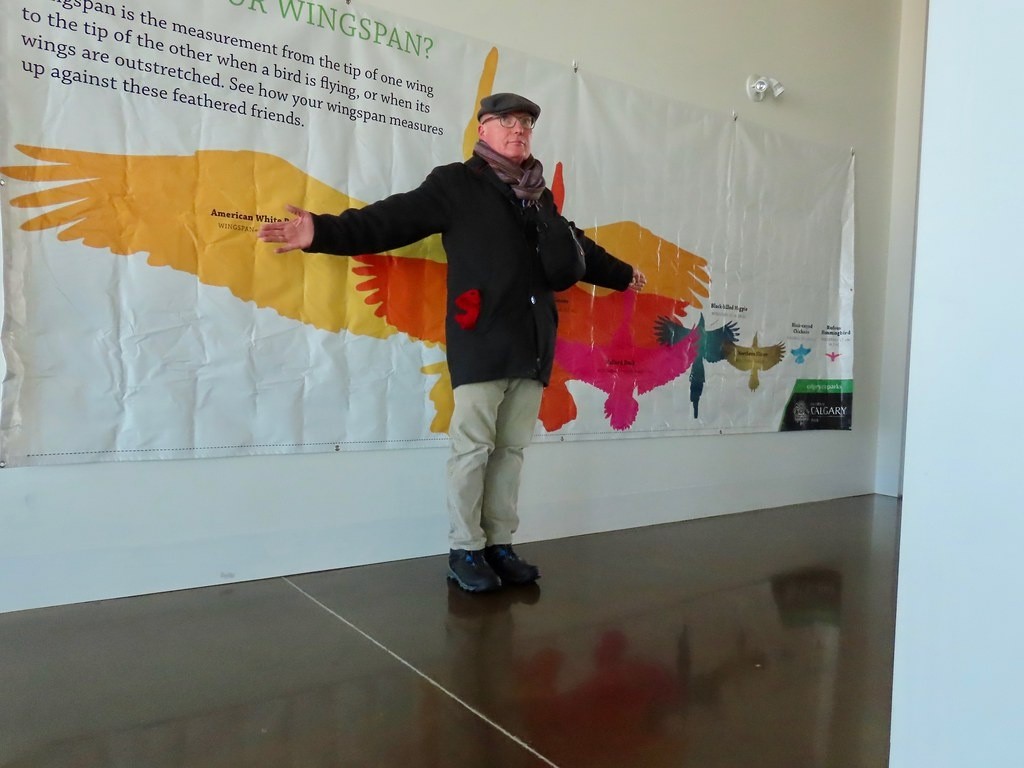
[448,548,498,591]
[482,543,541,587]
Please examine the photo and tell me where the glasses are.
[481,112,536,130]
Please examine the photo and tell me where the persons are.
[258,92,647,591]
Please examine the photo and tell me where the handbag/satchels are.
[536,215,586,293]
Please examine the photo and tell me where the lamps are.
[746,73,785,102]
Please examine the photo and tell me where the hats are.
[477,93,541,126]
